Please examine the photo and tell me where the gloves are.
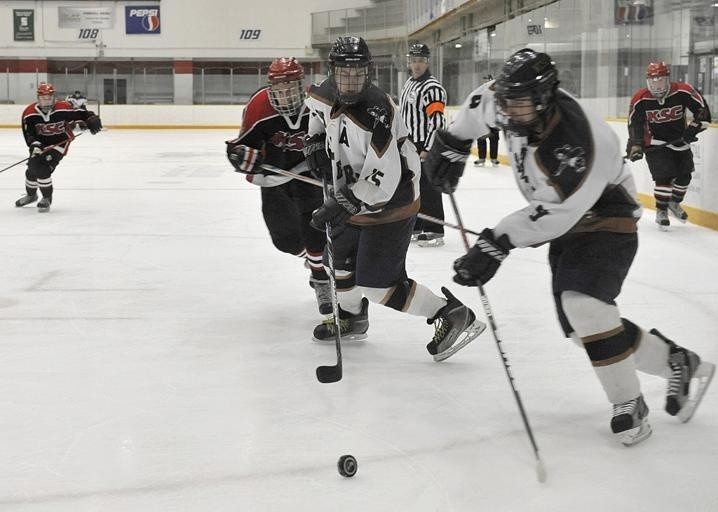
[424,129,472,194]
[236,146,263,172]
[683,121,706,144]
[452,228,516,286]
[303,133,332,184]
[86,115,102,135]
[31,147,44,160]
[627,138,643,161]
[309,185,362,233]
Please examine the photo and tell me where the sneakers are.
[669,201,688,220]
[491,159,499,165]
[418,231,444,239]
[38,197,51,207]
[314,297,369,340]
[313,281,333,314]
[650,328,700,416]
[611,395,649,433]
[656,207,669,225]
[427,287,476,354]
[474,159,485,164]
[16,194,38,206]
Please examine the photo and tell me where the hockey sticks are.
[316,170,343,381]
[444,178,546,483]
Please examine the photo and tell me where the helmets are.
[328,35,375,106]
[488,49,561,135]
[37,84,56,110]
[266,56,306,117]
[407,44,431,70]
[646,62,670,98]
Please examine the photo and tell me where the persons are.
[67,89,87,133]
[423,48,701,433]
[225,57,337,320]
[400,43,444,239]
[471,73,502,164]
[303,32,475,355]
[623,60,710,225]
[15,83,103,208]
